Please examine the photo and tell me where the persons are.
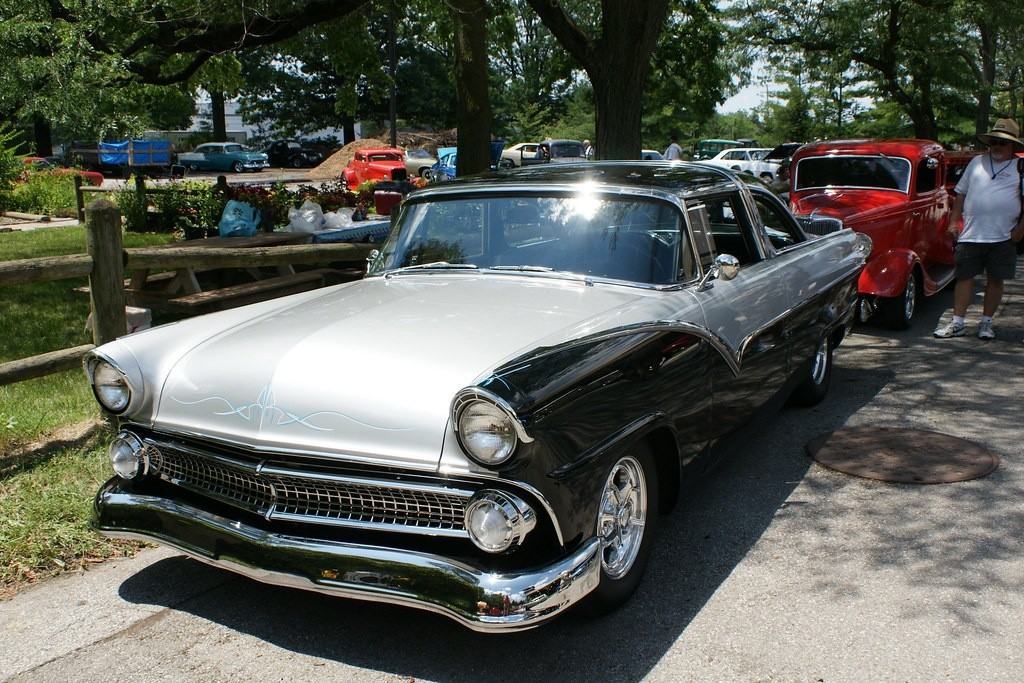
[212,175,232,191]
[932,118,1024,339]
[663,136,682,160]
[583,140,594,158]
[534,147,549,159]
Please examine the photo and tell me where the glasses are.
[988,139,1010,147]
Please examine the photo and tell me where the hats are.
[976,118,1024,152]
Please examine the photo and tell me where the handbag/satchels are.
[218,199,262,237]
[1016,157,1024,256]
[285,198,332,233]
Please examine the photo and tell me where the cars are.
[944,148,1023,256]
[430,152,457,181]
[641,138,804,192]
[521,139,587,166]
[260,139,323,169]
[340,149,408,191]
[496,142,547,179]
[15,157,104,187]
[83,160,873,636]
[398,148,438,182]
[786,137,968,330]
[177,142,269,173]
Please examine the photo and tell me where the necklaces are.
[989,153,1012,180]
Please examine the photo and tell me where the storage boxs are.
[374,190,401,215]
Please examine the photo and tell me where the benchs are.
[712,231,792,267]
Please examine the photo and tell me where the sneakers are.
[978,322,995,340]
[933,321,966,338]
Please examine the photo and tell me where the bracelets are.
[948,226,959,231]
